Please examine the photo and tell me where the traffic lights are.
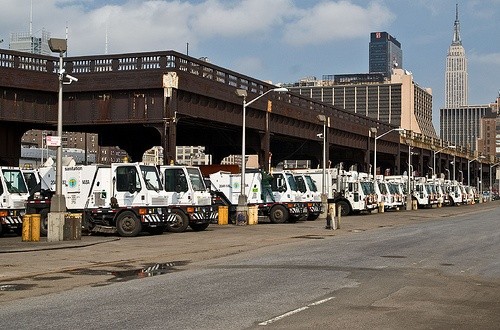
[267,100,273,113]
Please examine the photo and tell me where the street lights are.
[234,87,288,206]
[47,38,67,212]
[317,114,327,203]
[430,146,456,176]
[371,127,404,181]
[464,155,486,194]
[404,138,412,199]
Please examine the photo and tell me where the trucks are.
[0,156,500,237]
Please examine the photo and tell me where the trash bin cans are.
[246,206,258,224]
[379,202,384,212]
[66,213,83,239]
[23,214,40,242]
[218,206,228,225]
[413,200,417,210]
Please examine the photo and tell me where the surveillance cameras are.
[172,117,176,123]
[317,133,323,136]
[65,74,78,82]
